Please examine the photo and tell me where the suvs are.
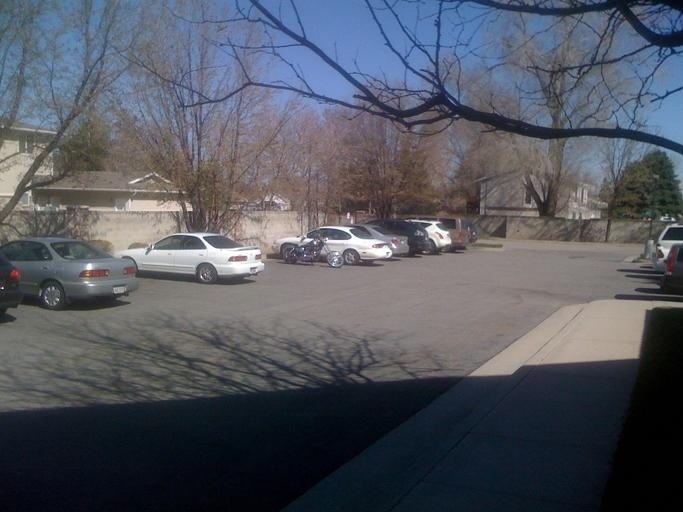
[660,244,683,295]
[651,223,683,273]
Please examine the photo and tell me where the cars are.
[1,235,141,311]
[271,211,481,268]
[0,250,24,317]
[113,230,268,284]
